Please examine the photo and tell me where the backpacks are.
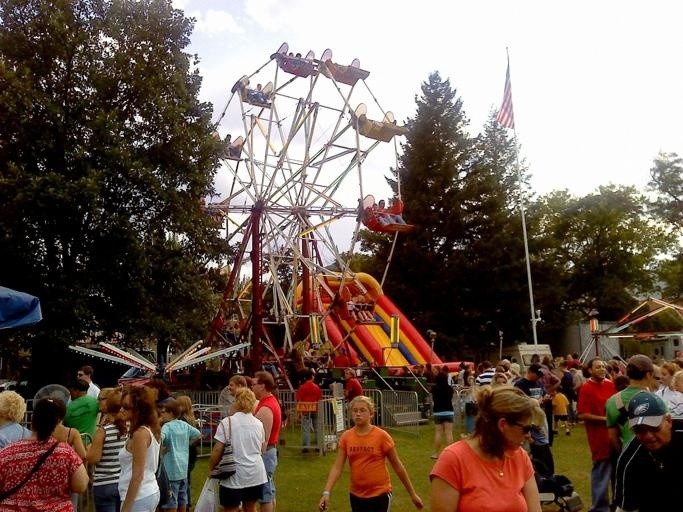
[535,474,574,497]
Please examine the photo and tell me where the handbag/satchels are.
[210,439,236,478]
[155,473,173,506]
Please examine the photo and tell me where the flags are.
[493,47,517,133]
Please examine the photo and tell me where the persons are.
[203,52,411,353]
[0,352,682,512]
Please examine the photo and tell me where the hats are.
[628,391,668,430]
[627,355,655,372]
[529,363,544,377]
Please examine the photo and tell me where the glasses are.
[77,372,85,377]
[652,376,662,381]
[120,402,133,410]
[97,396,107,401]
[514,422,535,433]
